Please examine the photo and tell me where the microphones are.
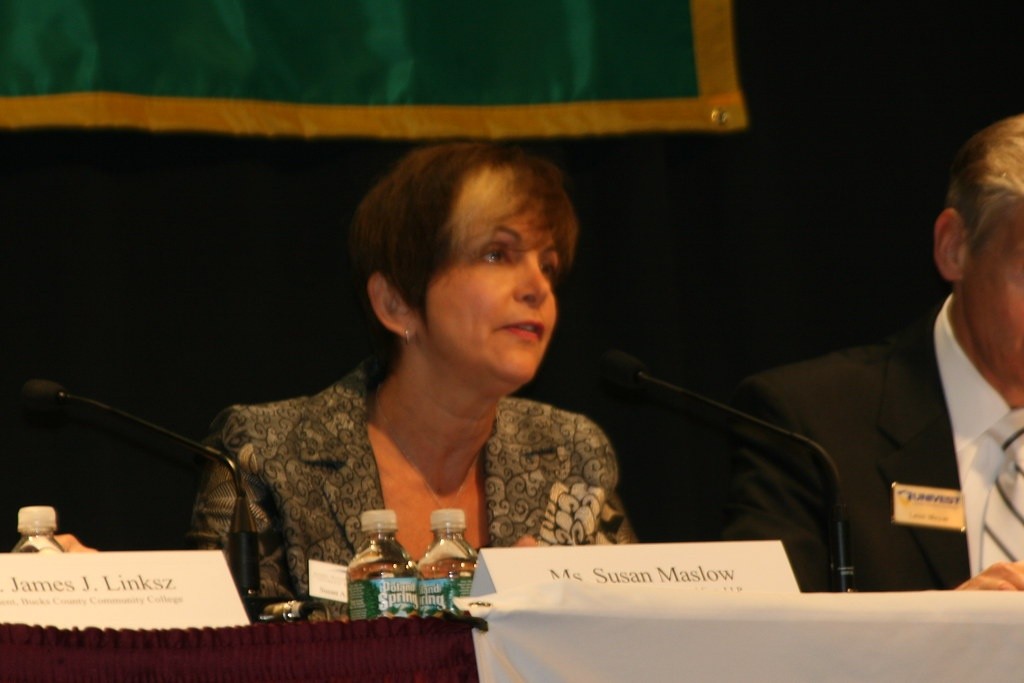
[19,379,288,622]
[600,349,852,593]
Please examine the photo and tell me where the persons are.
[677,111,1024,592]
[177,139,643,620]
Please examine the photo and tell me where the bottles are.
[347,509,418,619]
[10,506,64,553]
[417,509,480,617]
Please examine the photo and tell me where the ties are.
[980,408,1024,570]
